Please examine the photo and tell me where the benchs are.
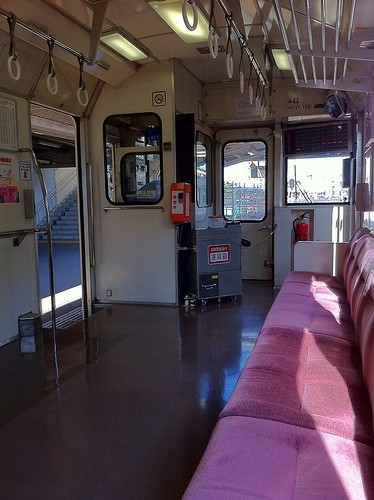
[183,226,374,500]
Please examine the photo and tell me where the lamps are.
[100,30,150,62]
[268,44,293,71]
[148,0,221,44]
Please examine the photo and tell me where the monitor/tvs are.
[326,94,343,118]
